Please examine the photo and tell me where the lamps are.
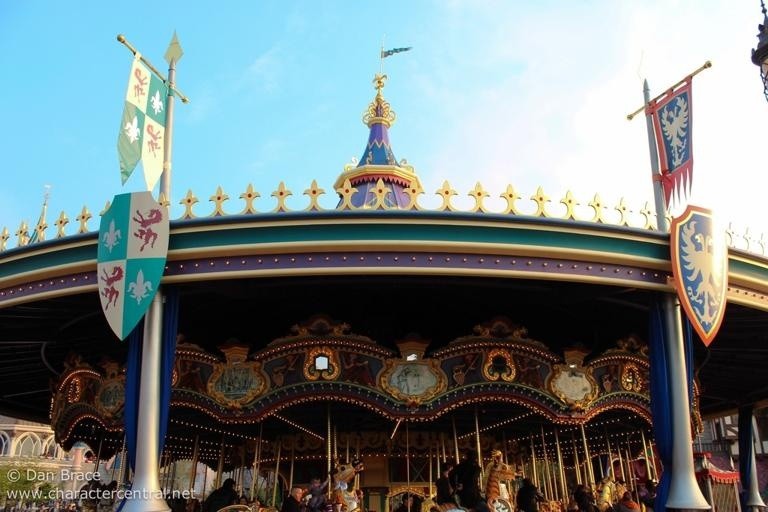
[313,352,329,380]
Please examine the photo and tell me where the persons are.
[391,461,659,512]
[4,477,117,512]
[160,478,379,511]
